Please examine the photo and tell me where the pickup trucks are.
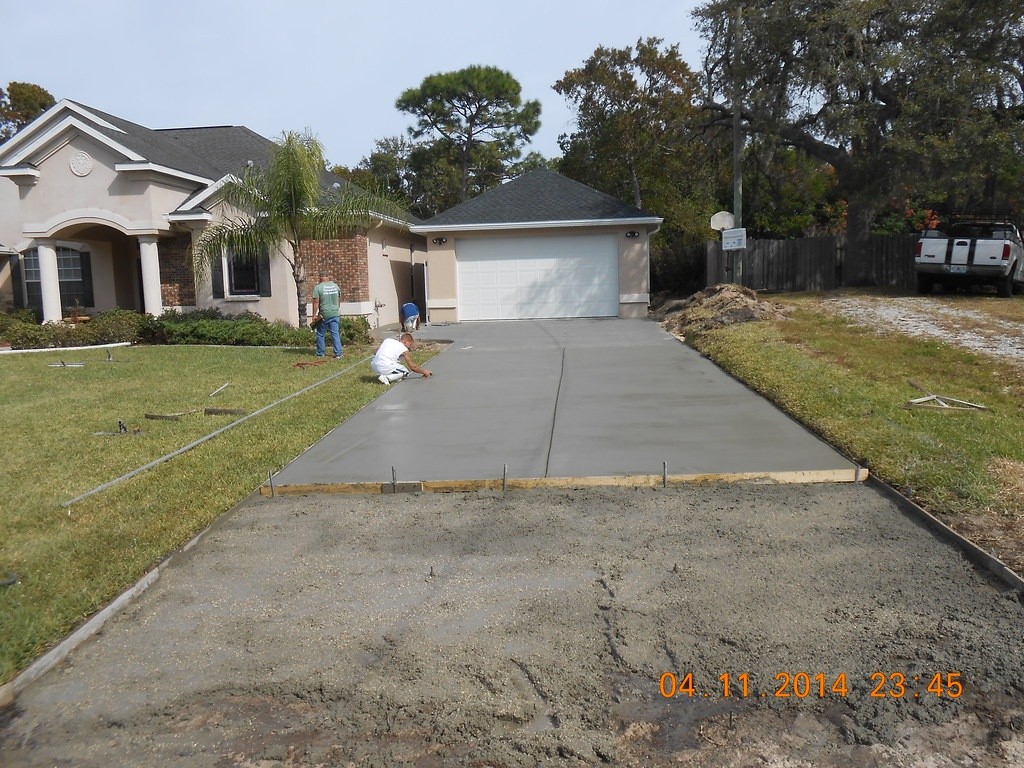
[913,219,1024,298]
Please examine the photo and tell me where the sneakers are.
[378,375,390,385]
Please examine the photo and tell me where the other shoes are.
[315,352,324,357]
[333,355,343,359]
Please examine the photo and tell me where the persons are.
[371,333,432,386]
[312,272,344,359]
[399,302,421,333]
